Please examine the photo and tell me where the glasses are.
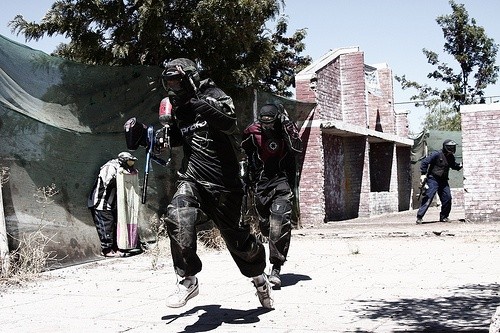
[162,75,183,93]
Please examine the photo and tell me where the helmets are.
[118,152,138,169]
[443,140,458,154]
[260,105,279,121]
[162,58,200,103]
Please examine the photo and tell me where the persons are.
[124,58,272,307]
[241,104,303,287]
[416,139,463,223]
[88,152,138,257]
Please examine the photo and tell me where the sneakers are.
[258,217,269,236]
[269,264,281,286]
[167,279,199,307]
[252,274,274,310]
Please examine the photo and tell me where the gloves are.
[420,175,427,184]
[245,124,262,135]
[186,76,197,102]
[138,124,149,145]
[285,121,293,134]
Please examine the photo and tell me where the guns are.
[239,155,256,214]
[416,162,432,201]
[124,117,168,204]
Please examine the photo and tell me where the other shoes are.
[416,216,422,224]
[440,217,448,222]
[99,249,129,257]
